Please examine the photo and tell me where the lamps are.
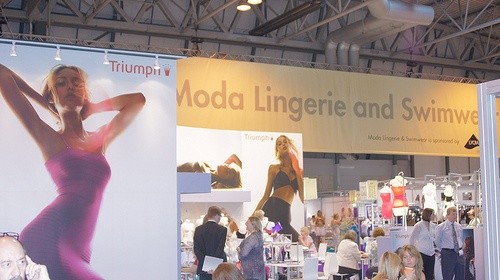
[237,0,251,11]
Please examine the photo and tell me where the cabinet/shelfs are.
[263,234,303,280]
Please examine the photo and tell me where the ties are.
[451,224,459,254]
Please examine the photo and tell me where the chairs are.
[303,258,329,280]
[318,243,327,275]
[327,252,348,280]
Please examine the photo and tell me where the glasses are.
[0,232,19,240]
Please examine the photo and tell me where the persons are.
[411,194,426,211]
[180,219,195,245]
[211,261,246,280]
[423,183,437,214]
[235,217,266,280]
[193,214,209,266]
[245,209,264,237]
[193,206,228,280]
[466,207,482,227]
[379,185,395,220]
[253,134,304,242]
[177,152,242,190]
[340,206,353,220]
[0,232,51,279]
[365,226,385,279]
[398,244,426,280]
[443,184,455,214]
[435,206,464,280]
[409,208,437,280]
[297,226,318,256]
[0,63,146,280]
[390,175,409,216]
[336,229,362,280]
[370,249,402,280]
[309,210,342,230]
